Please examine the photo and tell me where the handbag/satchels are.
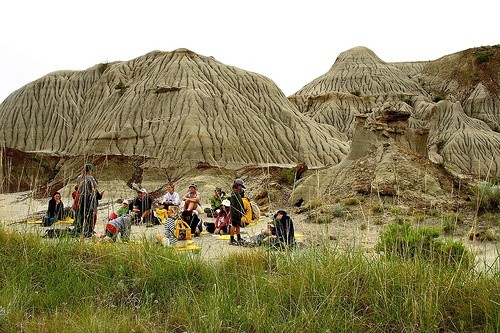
[40,229,60,238]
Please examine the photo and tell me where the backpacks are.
[243,197,260,222]
[167,217,190,239]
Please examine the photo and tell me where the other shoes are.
[228,240,238,246]
[237,239,248,246]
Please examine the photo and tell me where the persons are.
[292,161,308,185]
[41,163,296,252]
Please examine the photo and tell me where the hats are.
[189,183,198,188]
[221,199,230,206]
[274,210,287,218]
[234,179,245,187]
[138,188,146,193]
[86,163,94,169]
[214,186,222,191]
[123,200,128,204]
